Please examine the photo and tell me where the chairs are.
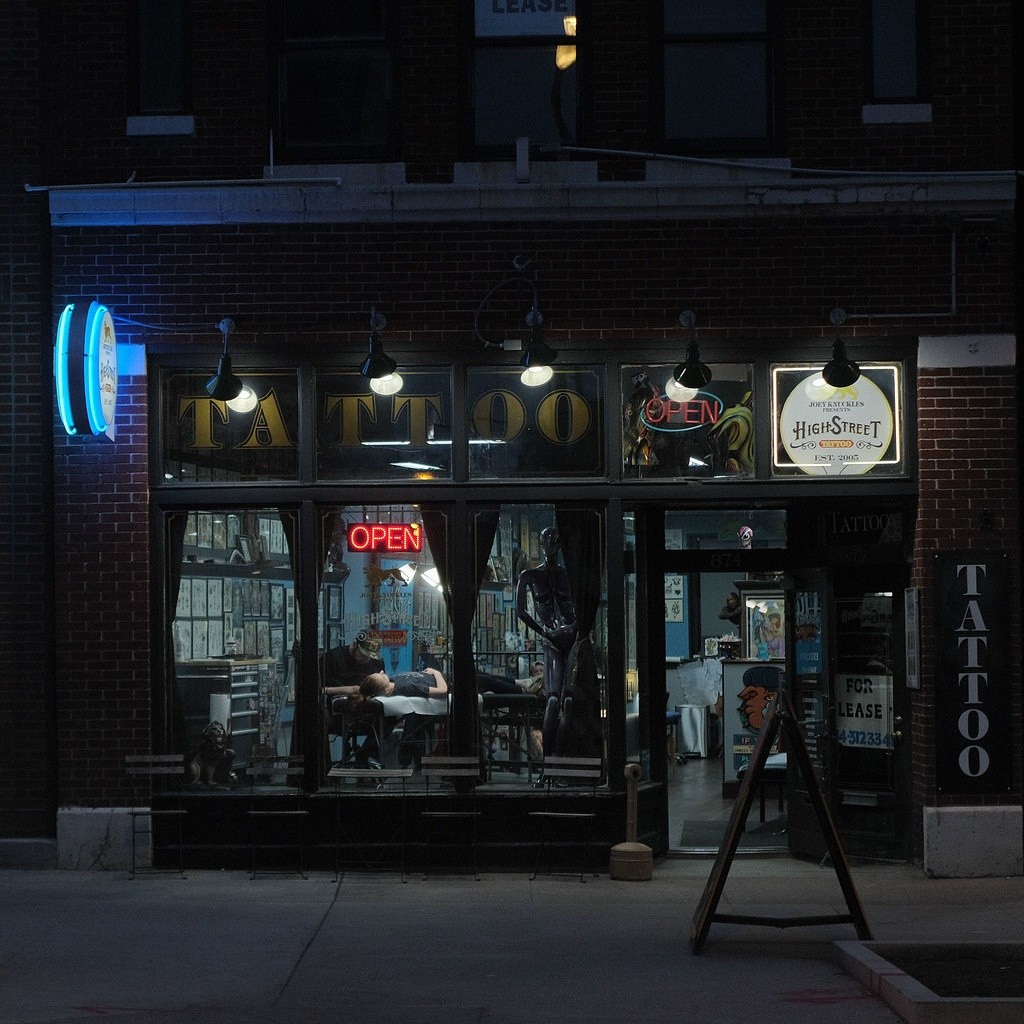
[245,755,309,880]
[528,757,602,884]
[736,672,788,823]
[122,754,189,880]
[420,757,482,883]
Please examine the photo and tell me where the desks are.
[326,767,414,884]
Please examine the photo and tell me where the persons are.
[718,592,742,625]
[528,661,544,677]
[358,667,545,697]
[319,628,400,770]
[515,527,580,754]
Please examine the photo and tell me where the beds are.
[333,688,538,787]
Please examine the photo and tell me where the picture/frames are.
[740,590,785,659]
[173,511,344,707]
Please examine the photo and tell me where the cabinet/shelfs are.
[176,657,261,786]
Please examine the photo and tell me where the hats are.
[356,628,381,660]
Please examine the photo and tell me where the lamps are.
[666,376,699,402]
[361,303,397,378]
[369,373,403,396]
[520,306,558,368]
[823,328,862,388]
[520,366,554,386]
[225,386,258,413]
[675,328,711,388]
[202,319,242,401]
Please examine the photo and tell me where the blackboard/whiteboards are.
[690,687,874,957]
